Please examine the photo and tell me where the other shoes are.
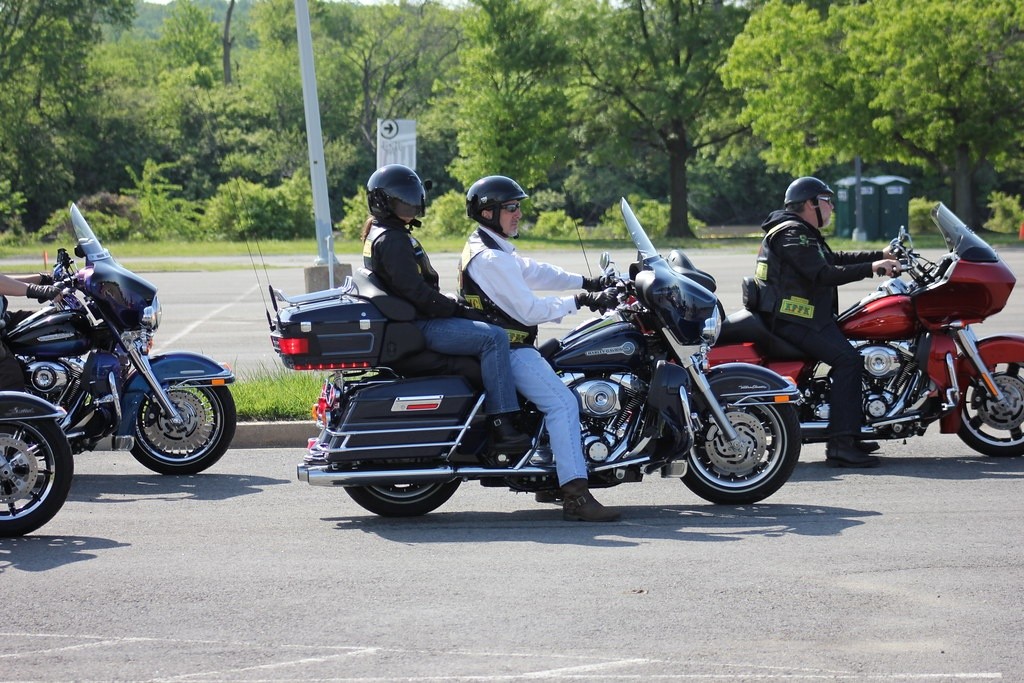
[825,444,879,467]
[857,441,880,453]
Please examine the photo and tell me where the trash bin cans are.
[833,176,880,241]
[870,175,910,240]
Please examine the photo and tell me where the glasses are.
[818,196,831,205]
[487,203,521,213]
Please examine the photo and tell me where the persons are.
[754,174,903,468]
[362,164,531,447]
[0,272,64,482]
[459,176,622,522]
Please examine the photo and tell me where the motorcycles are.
[0,391,75,538]
[8,201,238,475]
[619,200,1024,456]
[267,196,805,517]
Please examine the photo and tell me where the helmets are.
[367,164,426,230]
[466,175,529,217]
[784,176,834,204]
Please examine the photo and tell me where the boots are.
[563,478,620,521]
[486,412,530,447]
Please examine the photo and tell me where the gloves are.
[582,275,605,292]
[576,288,620,310]
[457,299,490,323]
